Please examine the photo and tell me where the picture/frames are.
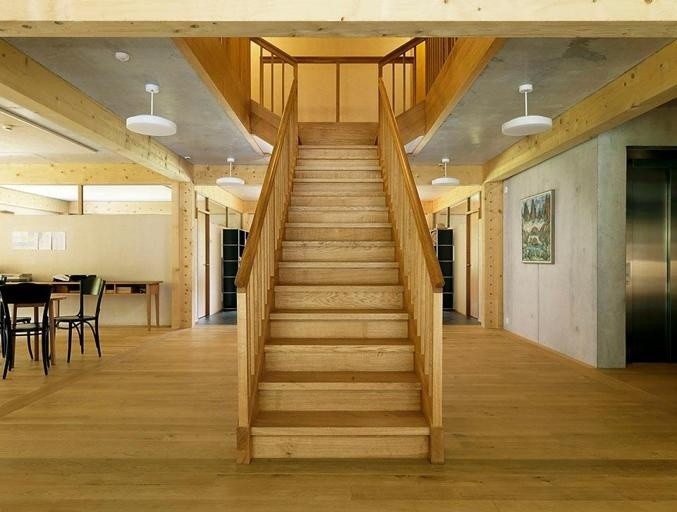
[521,190,555,264]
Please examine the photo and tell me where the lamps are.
[127,84,247,191]
[430,84,552,187]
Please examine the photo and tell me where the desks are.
[104,279,163,331]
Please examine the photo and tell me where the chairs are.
[1,277,105,379]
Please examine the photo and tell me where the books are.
[116,286,133,294]
[105,289,113,293]
